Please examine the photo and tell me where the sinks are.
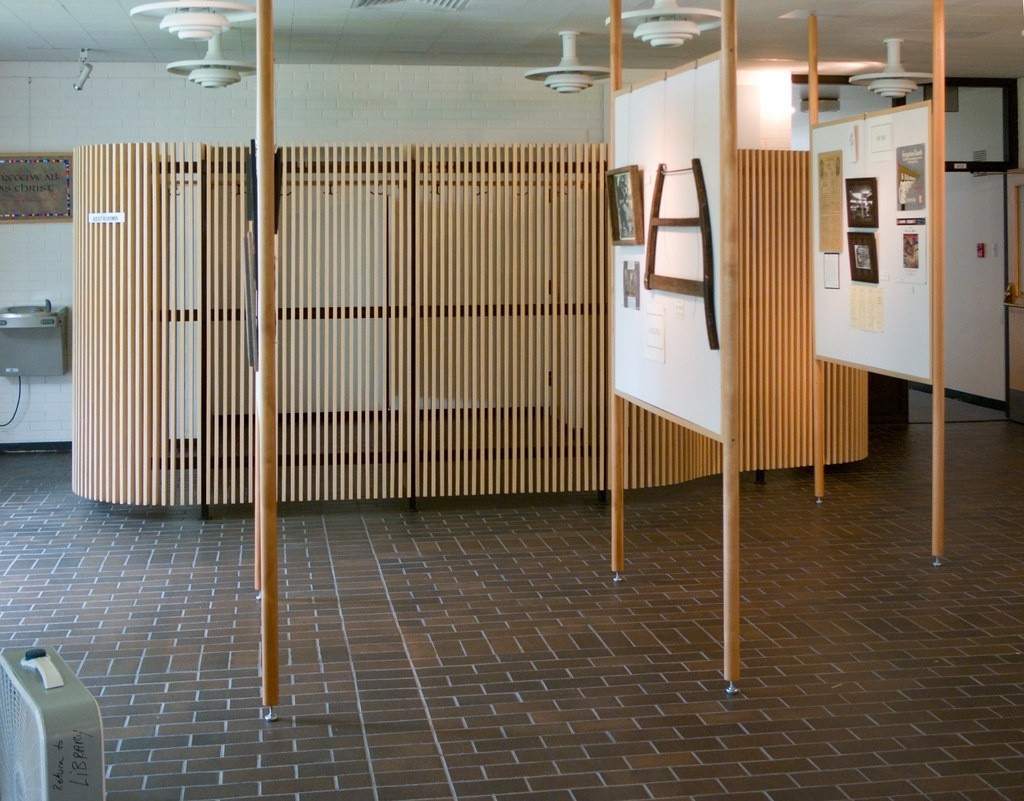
[7,306,44,314]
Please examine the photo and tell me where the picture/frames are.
[847,232,879,284]
[846,177,879,228]
[605,165,644,246]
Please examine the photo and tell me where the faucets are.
[46,299,52,312]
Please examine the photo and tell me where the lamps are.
[129,0,257,41]
[523,30,610,94]
[848,39,933,98]
[801,99,841,111]
[605,0,722,48]
[73,48,93,91]
[166,33,256,88]
